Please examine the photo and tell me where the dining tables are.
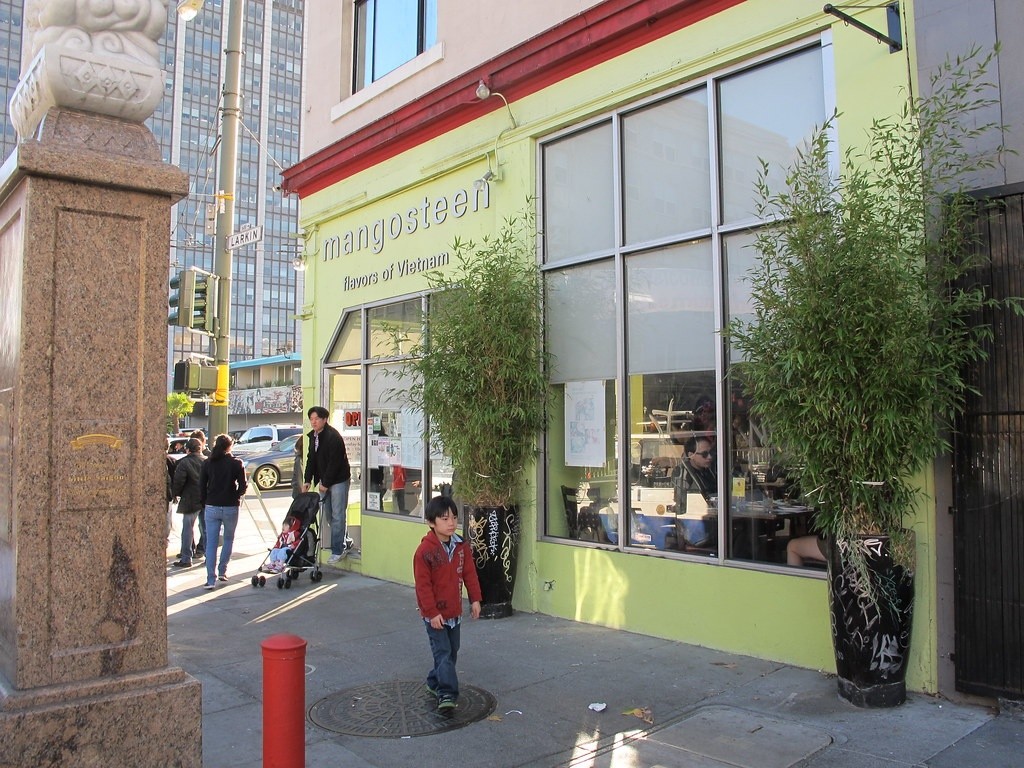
[695,496,819,561]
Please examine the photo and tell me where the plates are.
[778,505,806,511]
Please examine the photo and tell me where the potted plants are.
[371,191,564,622]
[712,25,1022,710]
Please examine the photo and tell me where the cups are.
[762,490,773,514]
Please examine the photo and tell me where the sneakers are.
[327,537,355,565]
[426,684,457,710]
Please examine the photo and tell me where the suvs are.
[237,423,303,453]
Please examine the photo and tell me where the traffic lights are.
[195,277,215,333]
[173,361,201,391]
[167,270,195,328]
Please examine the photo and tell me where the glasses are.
[696,449,715,458]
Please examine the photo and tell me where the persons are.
[785,533,830,570]
[648,461,655,488]
[172,438,208,567]
[303,406,353,563]
[370,412,422,516]
[176,430,211,558]
[165,433,178,563]
[670,435,762,561]
[262,516,303,573]
[693,389,801,502]
[170,442,186,454]
[291,435,303,499]
[199,434,247,590]
[411,495,483,711]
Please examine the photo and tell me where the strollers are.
[251,492,323,588]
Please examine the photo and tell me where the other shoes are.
[203,583,216,588]
[219,574,228,581]
[262,564,285,574]
[176,553,204,558]
[173,560,193,567]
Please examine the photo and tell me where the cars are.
[167,438,191,460]
[236,434,304,491]
